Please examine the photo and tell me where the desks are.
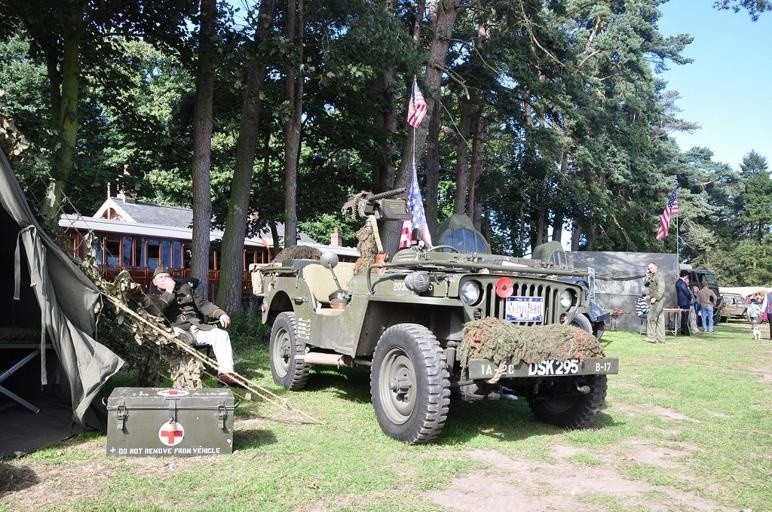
[639,308,689,337]
[0,327,55,416]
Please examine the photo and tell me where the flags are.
[406,75,429,129]
[656,189,679,241]
[399,166,433,249]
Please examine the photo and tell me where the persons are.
[145,266,247,388]
[747,291,772,339]
[675,270,717,335]
[636,263,668,343]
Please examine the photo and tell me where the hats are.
[679,270,689,275]
[152,264,173,278]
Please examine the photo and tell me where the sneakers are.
[645,337,665,343]
[217,373,248,389]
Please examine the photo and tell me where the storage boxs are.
[102,387,235,458]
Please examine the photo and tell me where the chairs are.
[139,278,221,386]
[303,263,356,303]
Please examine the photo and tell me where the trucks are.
[678,263,723,325]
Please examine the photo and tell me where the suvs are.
[718,291,764,323]
[247,186,622,444]
